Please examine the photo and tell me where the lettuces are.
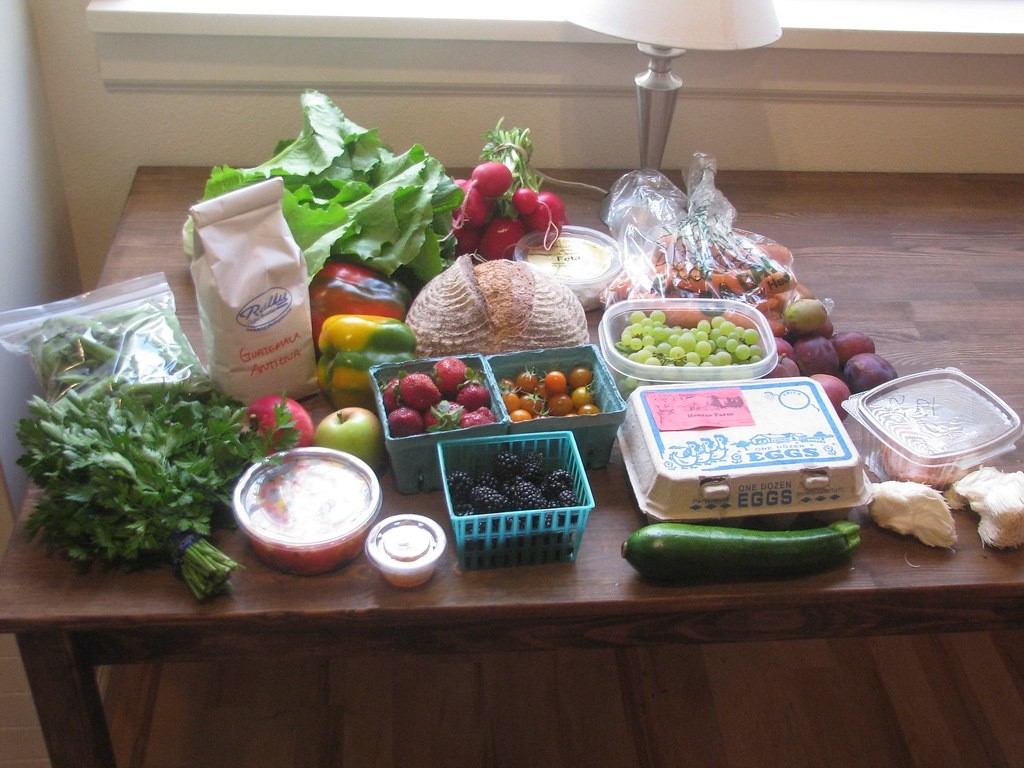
[181,91,462,283]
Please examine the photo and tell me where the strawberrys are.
[380,357,499,440]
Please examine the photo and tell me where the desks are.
[0,163,1024,767]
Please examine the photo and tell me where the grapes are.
[614,308,769,393]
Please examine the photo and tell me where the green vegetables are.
[15,370,305,599]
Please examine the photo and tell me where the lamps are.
[562,1,787,231]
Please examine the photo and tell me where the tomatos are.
[500,365,606,423]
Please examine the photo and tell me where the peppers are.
[306,256,417,410]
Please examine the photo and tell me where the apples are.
[239,395,387,473]
